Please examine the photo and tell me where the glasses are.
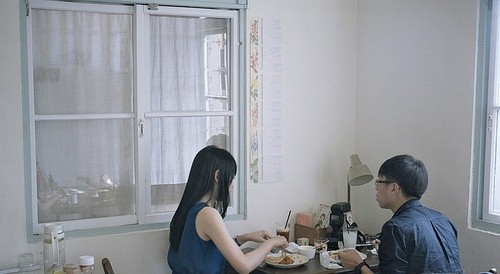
[375,178,400,191]
[232,177,236,182]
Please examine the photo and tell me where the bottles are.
[80,255,95,274]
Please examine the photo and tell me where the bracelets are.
[233,236,241,246]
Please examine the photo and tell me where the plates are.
[322,251,367,264]
[263,254,309,268]
[76,191,86,193]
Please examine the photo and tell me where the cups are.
[343,227,357,249]
[276,222,290,243]
[67,192,77,205]
[314,238,327,253]
[19,254,34,274]
[98,190,109,201]
[39,252,48,272]
[297,238,309,246]
[297,245,316,259]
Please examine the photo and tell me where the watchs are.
[354,262,370,274]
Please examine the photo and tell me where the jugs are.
[43,223,65,274]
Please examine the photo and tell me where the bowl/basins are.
[356,244,373,252]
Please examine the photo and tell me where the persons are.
[38,173,62,223]
[166,145,289,274]
[338,153,464,274]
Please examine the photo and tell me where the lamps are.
[348,153,374,217]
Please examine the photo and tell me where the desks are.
[225,238,380,274]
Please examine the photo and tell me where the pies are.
[264,257,283,263]
